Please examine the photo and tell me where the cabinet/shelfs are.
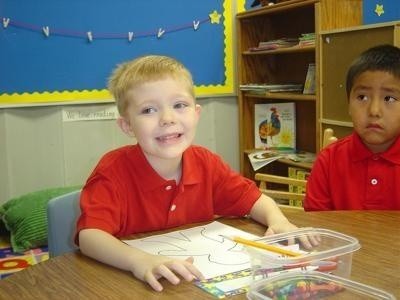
[237,1,363,209]
[317,20,400,155]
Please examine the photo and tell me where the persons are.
[304,45,400,210]
[74,55,322,292]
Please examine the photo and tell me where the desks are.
[0,209,400,300]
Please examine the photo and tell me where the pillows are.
[0,183,88,254]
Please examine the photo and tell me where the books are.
[240,33,316,171]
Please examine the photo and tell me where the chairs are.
[46,189,87,260]
[252,170,309,211]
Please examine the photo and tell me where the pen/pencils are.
[218,235,301,257]
